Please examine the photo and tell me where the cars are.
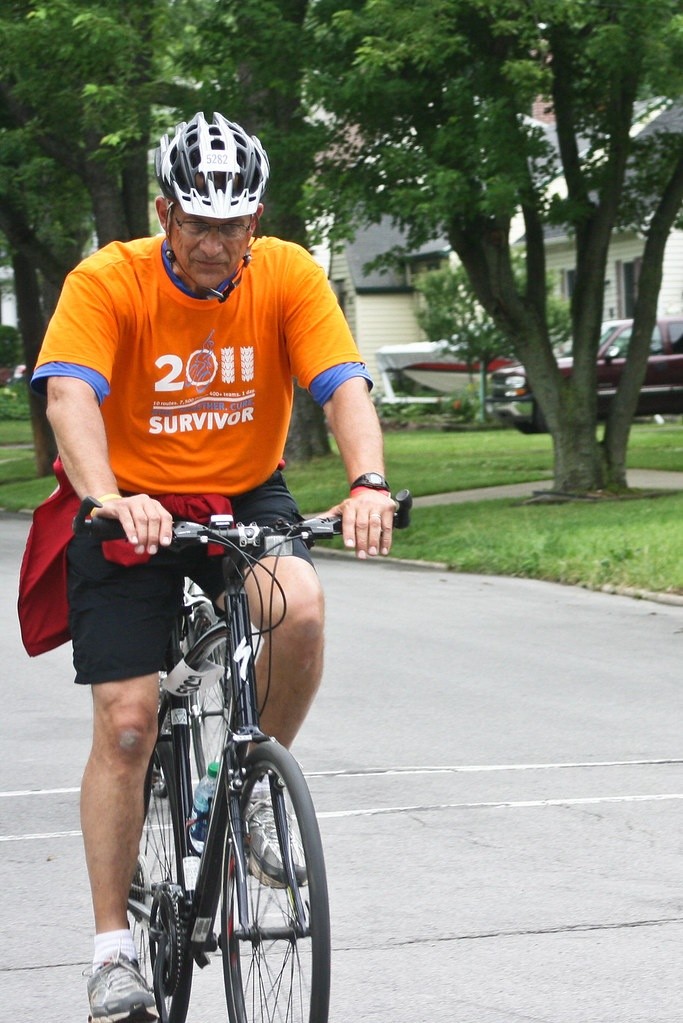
[484,317,683,434]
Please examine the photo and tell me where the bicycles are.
[70,479,412,1023]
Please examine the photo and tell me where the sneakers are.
[245,788,309,889]
[86,951,155,1023]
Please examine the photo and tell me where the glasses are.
[170,212,254,237]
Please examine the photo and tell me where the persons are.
[28,110,394,1023]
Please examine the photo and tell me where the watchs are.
[350,473,390,492]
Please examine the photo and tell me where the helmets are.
[156,111,269,220]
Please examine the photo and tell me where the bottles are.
[187,760,220,853]
